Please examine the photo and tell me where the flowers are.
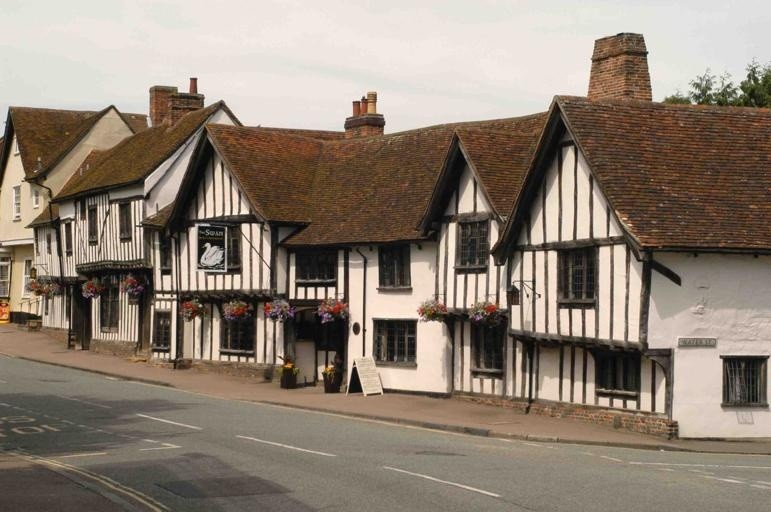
[180,297,348,327]
[417,299,447,322]
[466,301,501,328]
[324,359,343,383]
[26,276,142,298]
[279,354,298,375]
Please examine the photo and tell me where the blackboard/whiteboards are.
[354,356,382,395]
[152,309,172,350]
[195,223,227,273]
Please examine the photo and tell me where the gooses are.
[200,242,225,268]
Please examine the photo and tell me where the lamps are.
[506,280,533,305]
[30,264,48,279]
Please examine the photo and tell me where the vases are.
[323,371,342,392]
[280,367,296,388]
